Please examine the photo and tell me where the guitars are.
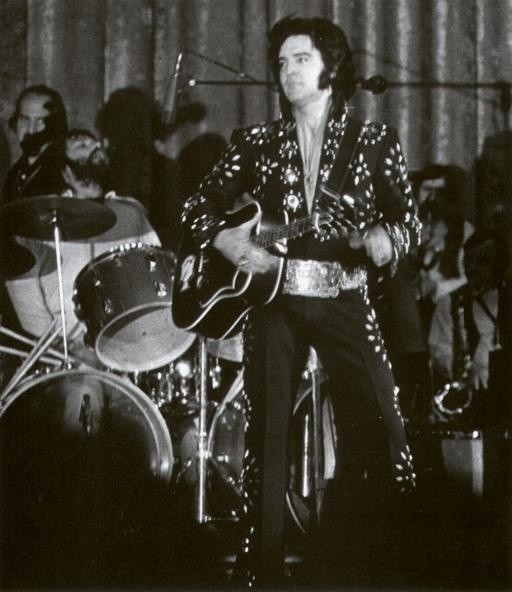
[172,197,353,342]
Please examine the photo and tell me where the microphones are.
[328,52,346,79]
[160,70,184,124]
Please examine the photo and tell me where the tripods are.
[166,337,255,528]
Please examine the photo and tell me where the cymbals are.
[0,242,34,279]
[0,196,115,240]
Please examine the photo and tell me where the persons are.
[178,14,426,591]
[2,87,511,567]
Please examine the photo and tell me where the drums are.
[0,366,174,559]
[72,243,196,374]
[158,398,245,536]
